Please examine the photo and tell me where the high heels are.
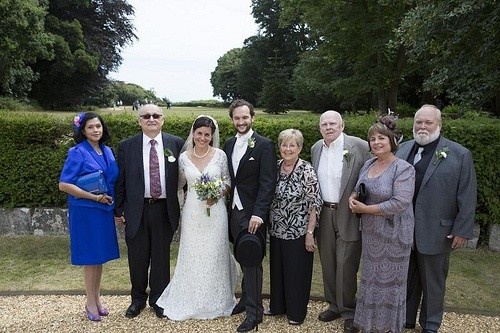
[85,303,101,321]
[98,307,109,316]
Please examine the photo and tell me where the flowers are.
[343,150,352,168]
[164,148,176,162]
[73,112,85,131]
[189,173,226,217]
[247,137,256,148]
[434,150,447,164]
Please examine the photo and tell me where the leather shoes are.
[153,304,166,319]
[318,310,340,322]
[344,319,358,333]
[231,303,245,315]
[125,303,146,318]
[236,318,262,332]
[405,322,415,329]
[421,330,437,333]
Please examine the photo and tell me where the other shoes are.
[264,308,273,316]
[289,319,302,324]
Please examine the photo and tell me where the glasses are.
[140,114,162,120]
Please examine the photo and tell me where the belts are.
[144,198,166,204]
[323,200,338,210]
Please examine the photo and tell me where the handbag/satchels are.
[355,183,366,219]
[73,170,108,201]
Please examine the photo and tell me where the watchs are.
[307,229,314,234]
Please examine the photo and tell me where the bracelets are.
[96,195,104,201]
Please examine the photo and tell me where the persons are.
[59,113,124,321]
[115,103,188,317]
[311,111,375,333]
[349,116,416,333]
[220,98,277,333]
[111,100,123,111]
[393,104,477,333]
[263,129,324,326]
[132,100,140,111]
[156,116,239,320]
[166,100,170,109]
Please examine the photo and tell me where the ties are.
[149,140,163,200]
[414,147,423,165]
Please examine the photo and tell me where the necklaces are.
[193,145,210,159]
[96,150,100,153]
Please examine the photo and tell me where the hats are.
[234,227,267,268]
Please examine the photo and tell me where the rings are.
[255,224,257,227]
